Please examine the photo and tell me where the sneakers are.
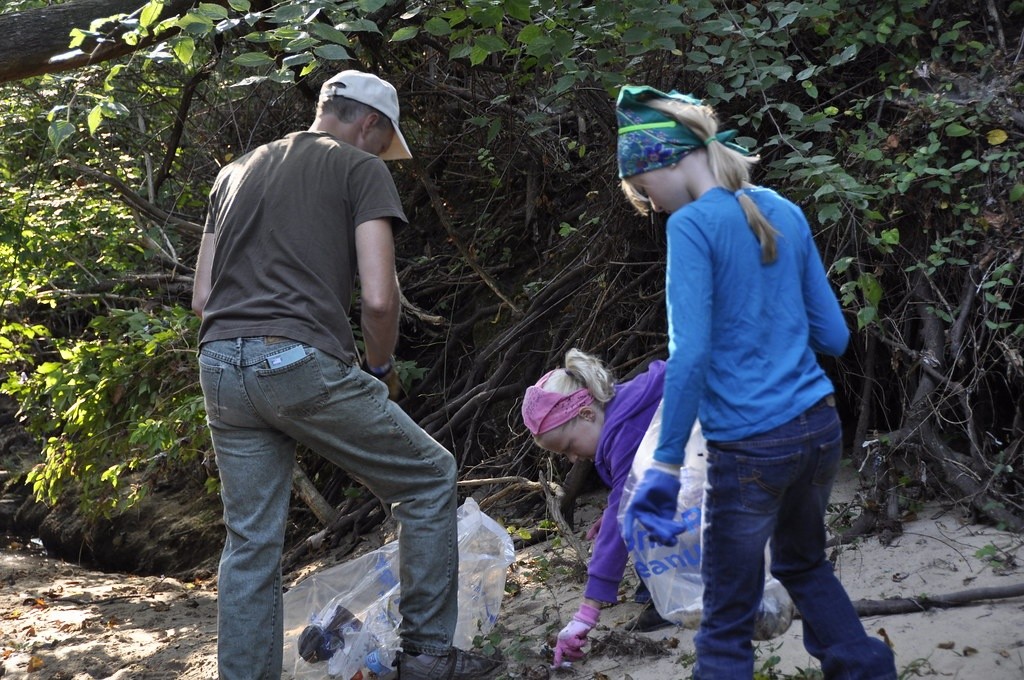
[397,647,507,680]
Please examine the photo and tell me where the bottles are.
[342,644,398,680]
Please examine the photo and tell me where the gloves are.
[554,603,600,667]
[624,460,687,546]
[586,517,603,541]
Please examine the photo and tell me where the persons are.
[615,83,899,680]
[521,348,709,668]
[191,71,508,680]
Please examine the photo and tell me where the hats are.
[321,71,413,161]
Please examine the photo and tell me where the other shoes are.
[625,603,674,632]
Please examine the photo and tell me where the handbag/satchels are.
[615,393,793,640]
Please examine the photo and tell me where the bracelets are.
[361,353,394,383]
